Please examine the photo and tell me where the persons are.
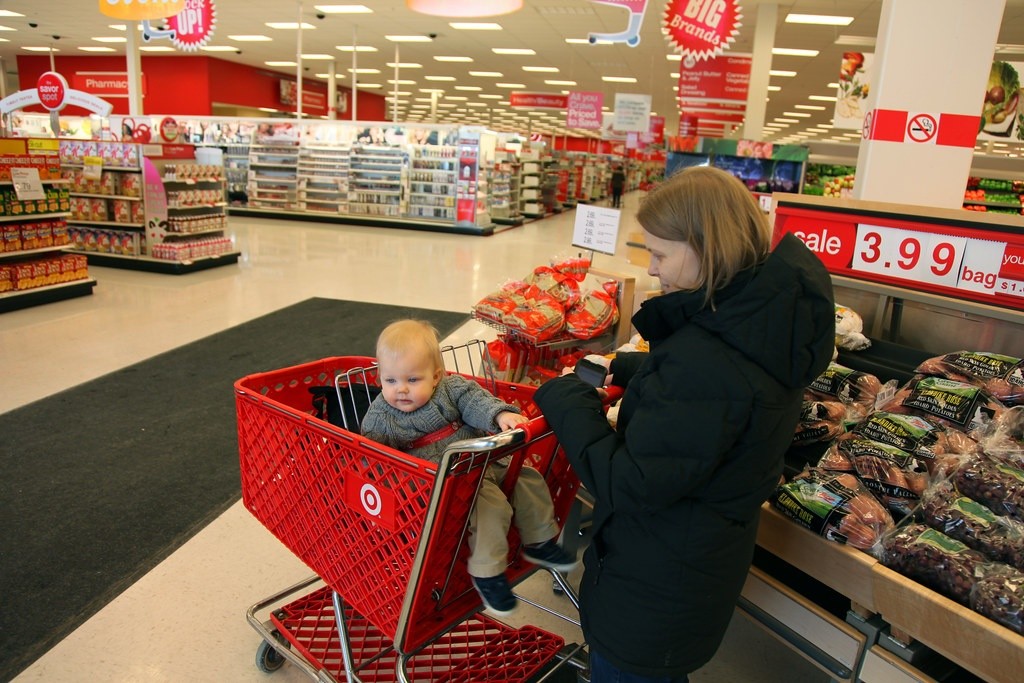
[611,166,626,208]
[258,124,272,136]
[122,124,131,141]
[532,166,836,683]
[414,128,432,145]
[361,318,581,616]
[217,124,236,144]
[369,127,381,144]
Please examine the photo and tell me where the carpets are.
[0,297,472,683]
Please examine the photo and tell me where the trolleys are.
[235,340,623,683]
[606,178,626,208]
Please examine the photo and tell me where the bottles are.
[416,148,454,157]
[150,236,234,260]
[167,213,226,233]
[167,190,178,207]
[411,172,454,181]
[414,159,442,169]
[163,163,221,178]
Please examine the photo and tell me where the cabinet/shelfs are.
[474,161,1024,683]
[193,120,648,237]
[0,137,98,315]
[58,138,241,274]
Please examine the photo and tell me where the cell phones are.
[573,359,608,388]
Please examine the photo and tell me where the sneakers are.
[471,572,519,616]
[520,538,581,571]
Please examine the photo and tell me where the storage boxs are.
[0,140,226,298]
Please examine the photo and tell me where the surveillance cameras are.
[29,23,37,27]
[304,67,309,70]
[236,51,241,54]
[53,36,60,39]
[316,15,325,20]
[428,34,437,37]
